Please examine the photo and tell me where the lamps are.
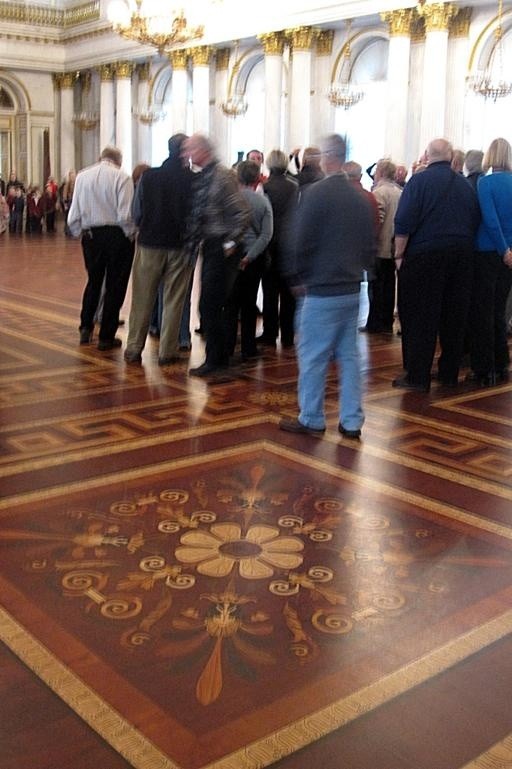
[132,58,168,127]
[326,22,367,106]
[103,0,209,57]
[221,39,250,118]
[71,66,101,132]
[468,0,511,103]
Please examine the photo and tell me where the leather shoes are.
[392,366,509,392]
[79,320,293,377]
[338,421,362,438]
[279,417,327,436]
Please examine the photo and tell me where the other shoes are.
[397,329,401,335]
[359,326,371,332]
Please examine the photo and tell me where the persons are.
[66,169,77,237]
[287,145,327,187]
[132,164,163,339]
[392,163,408,186]
[358,158,405,335]
[340,161,381,240]
[124,133,201,366]
[450,149,465,174]
[181,133,250,377]
[92,268,126,326]
[246,149,269,191]
[464,138,511,389]
[464,149,487,191]
[390,138,478,392]
[222,159,274,362]
[0,173,67,236]
[253,149,302,350]
[67,146,139,351]
[177,267,194,352]
[279,133,375,438]
